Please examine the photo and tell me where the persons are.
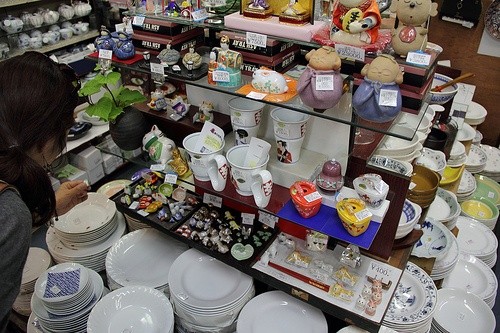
[246,0,272,11]
[1,49,90,330]
[349,55,405,121]
[94,29,243,89]
[294,47,343,110]
[280,0,308,15]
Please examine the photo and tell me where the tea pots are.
[0,0,97,89]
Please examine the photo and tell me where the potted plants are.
[78,63,146,151]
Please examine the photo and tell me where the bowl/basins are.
[368,73,459,260]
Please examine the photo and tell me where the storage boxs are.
[130,13,438,115]
[48,133,141,193]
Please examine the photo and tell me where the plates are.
[11,101,500,333]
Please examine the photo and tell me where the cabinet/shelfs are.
[85,9,476,333]
[0,0,109,159]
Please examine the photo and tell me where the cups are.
[269,105,310,163]
[227,95,266,147]
[226,144,273,209]
[182,132,228,192]
